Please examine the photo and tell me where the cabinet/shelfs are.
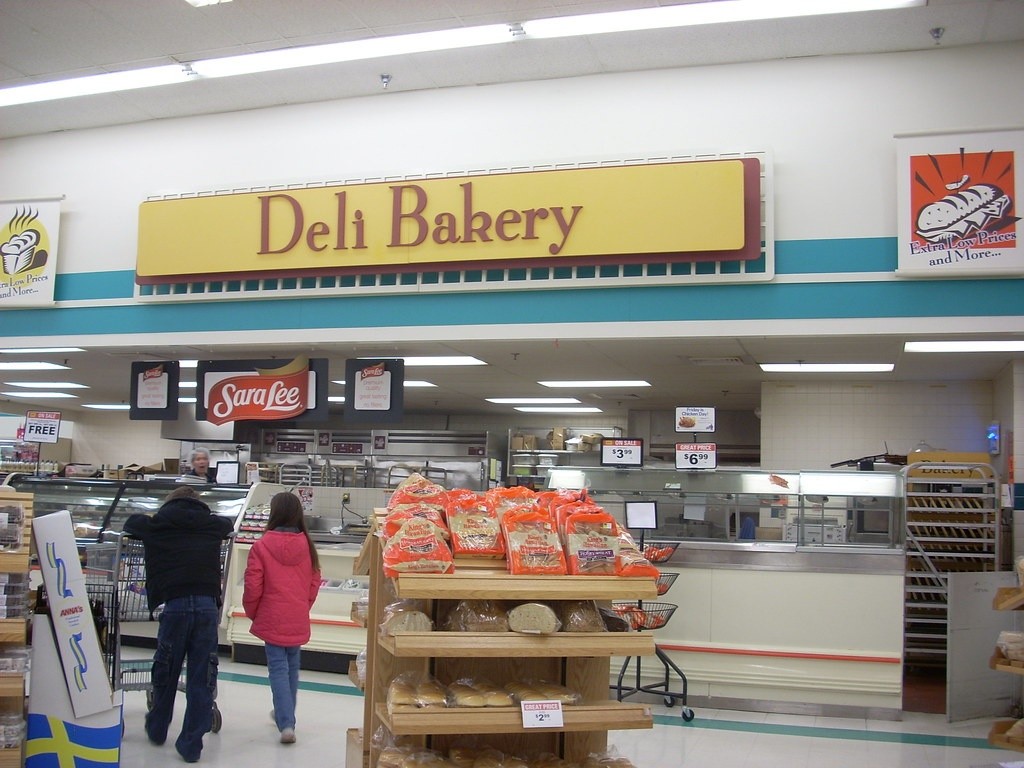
[900,463,1002,675]
[0,470,250,648]
[225,480,401,664]
[0,491,34,768]
[346,508,657,768]
[506,425,623,490]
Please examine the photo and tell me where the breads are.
[74,522,102,537]
[996,629,1024,662]
[354,595,638,768]
[1005,718,1024,737]
[1016,558,1024,591]
[679,416,695,427]
[385,476,657,577]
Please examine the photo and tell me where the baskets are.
[655,573,681,596]
[634,542,680,563]
[611,601,679,630]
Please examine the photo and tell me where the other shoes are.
[270,709,276,721]
[280,727,296,743]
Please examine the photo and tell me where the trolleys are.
[607,539,694,723]
[82,530,236,735]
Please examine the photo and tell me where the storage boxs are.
[783,523,846,544]
[511,427,603,476]
[102,463,143,479]
[905,451,993,478]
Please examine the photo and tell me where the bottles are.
[89,597,108,653]
[0,459,58,473]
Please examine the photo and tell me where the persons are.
[185,447,213,482]
[122,486,235,763]
[242,492,323,742]
